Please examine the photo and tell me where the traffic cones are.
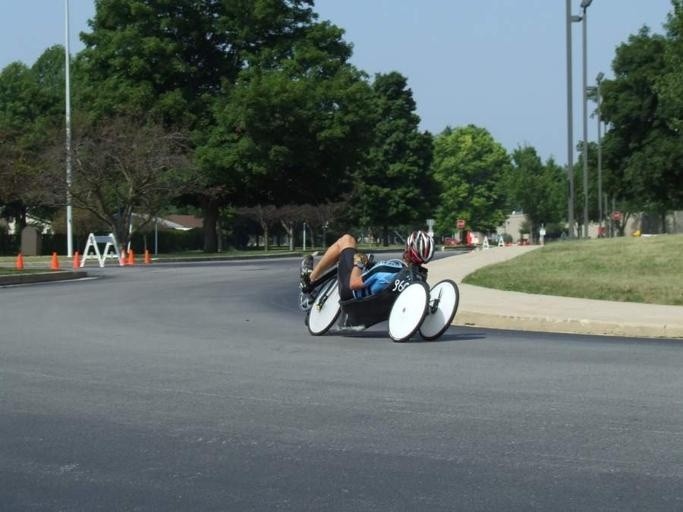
[120,249,151,266]
[50,252,59,271]
[73,251,80,269]
[16,252,23,272]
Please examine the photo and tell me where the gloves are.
[353,253,369,269]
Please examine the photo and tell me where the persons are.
[300,230,436,301]
[539,223,546,247]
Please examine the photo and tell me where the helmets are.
[406,231,435,265]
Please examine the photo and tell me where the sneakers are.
[300,254,313,294]
